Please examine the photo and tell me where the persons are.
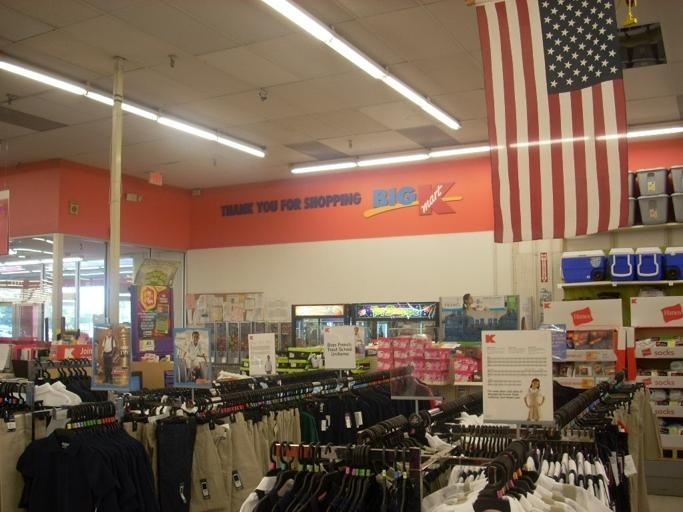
[461,293,475,311]
[352,327,362,353]
[99,327,116,384]
[181,331,207,373]
[524,378,545,421]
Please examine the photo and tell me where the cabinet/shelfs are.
[547,325,627,388]
[628,326,683,497]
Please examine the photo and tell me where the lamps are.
[261,0,461,131]
[0,52,266,158]
[288,121,683,176]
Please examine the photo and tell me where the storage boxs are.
[627,165,683,226]
[560,246,683,282]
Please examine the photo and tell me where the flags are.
[472,0,628,244]
